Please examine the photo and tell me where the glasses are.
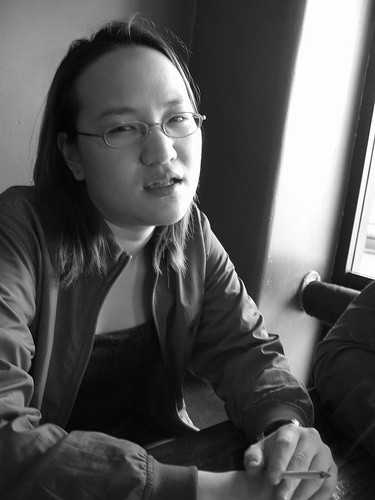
[77,113,206,148]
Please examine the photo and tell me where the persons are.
[0,13,339,500]
[311,281,375,458]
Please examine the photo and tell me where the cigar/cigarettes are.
[280,471,329,480]
